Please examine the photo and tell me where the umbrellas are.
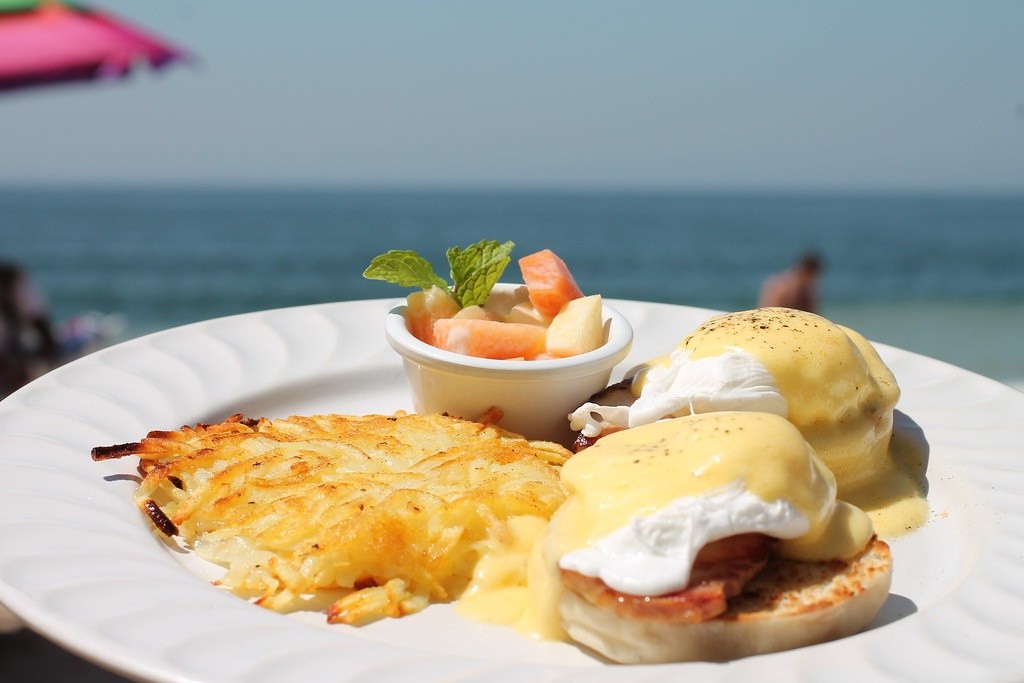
[0,0,173,91]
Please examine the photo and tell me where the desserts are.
[527,412,894,662]
[624,306,927,527]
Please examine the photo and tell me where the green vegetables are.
[363,240,514,309]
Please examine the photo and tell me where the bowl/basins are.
[384,283,634,448]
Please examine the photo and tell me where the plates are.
[0,288,1024,683]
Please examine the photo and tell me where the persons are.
[759,249,822,317]
[0,263,59,406]
